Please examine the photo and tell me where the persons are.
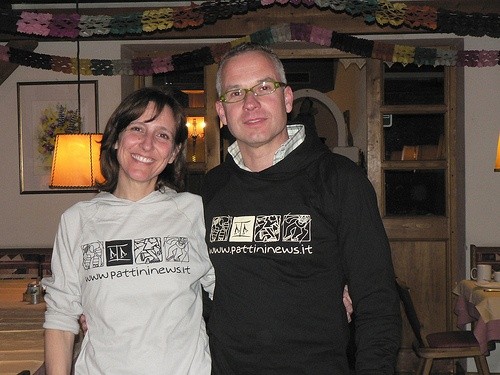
[78,41,404,375]
[39,86,354,375]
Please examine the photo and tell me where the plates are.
[476,284,500,290]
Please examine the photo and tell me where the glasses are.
[218,80,288,104]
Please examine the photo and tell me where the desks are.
[0,279,47,375]
[452,280,500,356]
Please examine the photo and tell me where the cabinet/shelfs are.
[121,36,467,375]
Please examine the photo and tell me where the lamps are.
[186,119,206,163]
[49,0,109,188]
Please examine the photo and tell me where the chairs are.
[470,245,500,279]
[0,260,43,279]
[395,275,496,375]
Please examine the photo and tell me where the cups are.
[471,265,491,281]
[494,272,500,281]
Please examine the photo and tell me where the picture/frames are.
[17,80,100,194]
